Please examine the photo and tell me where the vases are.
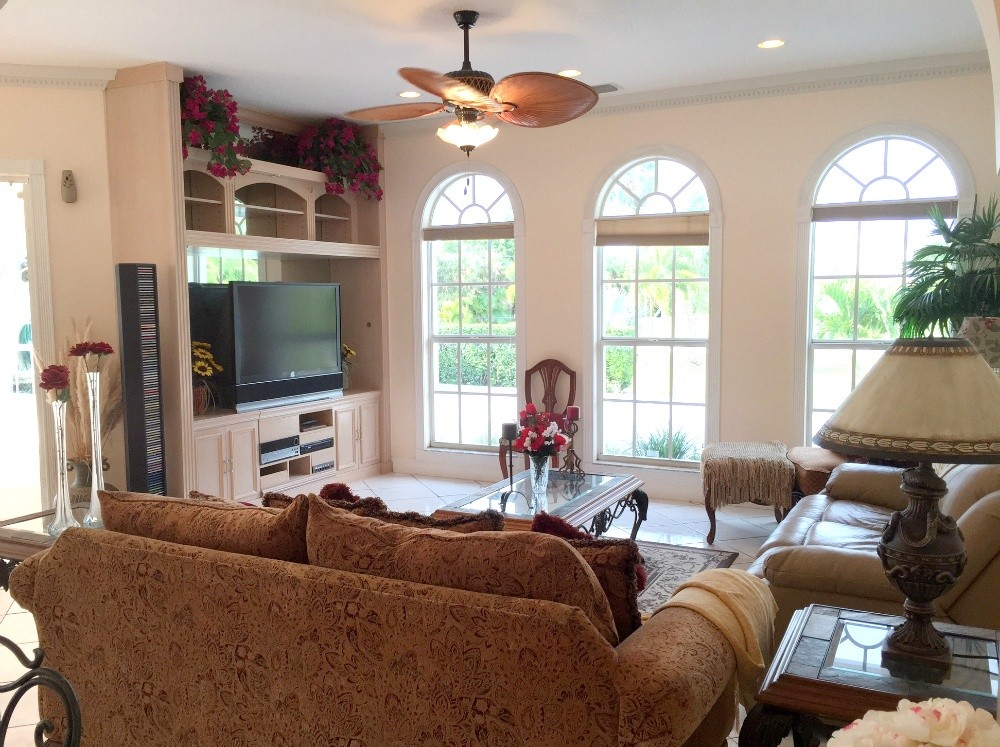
[526,455,550,516]
[82,372,106,529]
[341,361,350,390]
[46,400,82,539]
[192,384,207,416]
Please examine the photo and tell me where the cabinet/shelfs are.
[181,141,230,250]
[313,170,358,257]
[336,389,382,485]
[193,405,262,504]
[230,155,313,255]
[257,396,336,501]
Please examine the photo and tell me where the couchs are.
[744,462,1000,650]
[7,525,778,747]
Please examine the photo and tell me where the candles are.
[502,423,517,438]
[566,406,580,420]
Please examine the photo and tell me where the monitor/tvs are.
[229,281,343,414]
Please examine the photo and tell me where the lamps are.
[956,316,1000,374]
[435,107,500,157]
[813,337,1000,672]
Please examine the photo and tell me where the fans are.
[343,10,599,129]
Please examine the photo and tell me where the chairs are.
[497,358,578,479]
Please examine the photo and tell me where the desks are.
[735,602,1000,747]
[0,500,92,564]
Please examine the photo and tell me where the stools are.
[699,438,797,546]
[782,445,849,519]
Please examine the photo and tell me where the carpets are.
[589,532,742,615]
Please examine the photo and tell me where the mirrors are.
[187,245,333,403]
[233,119,301,167]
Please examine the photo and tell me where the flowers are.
[38,364,72,525]
[510,403,570,487]
[341,343,356,369]
[296,116,385,201]
[178,74,254,181]
[249,125,300,166]
[67,340,115,491]
[826,696,1000,747]
[191,338,224,417]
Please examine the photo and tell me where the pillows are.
[266,491,388,517]
[305,489,621,650]
[349,508,504,533]
[559,534,644,644]
[92,484,308,565]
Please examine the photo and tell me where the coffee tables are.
[436,468,650,541]
